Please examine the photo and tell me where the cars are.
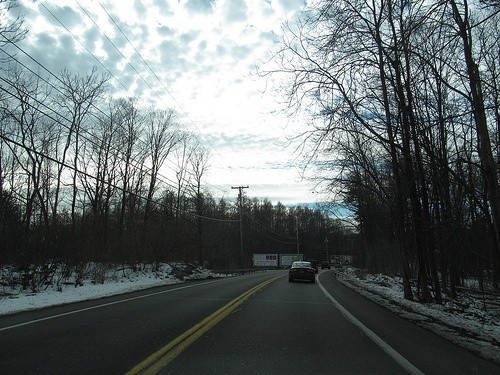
[321,261,330,269]
[289,262,316,283]
[307,260,318,273]
